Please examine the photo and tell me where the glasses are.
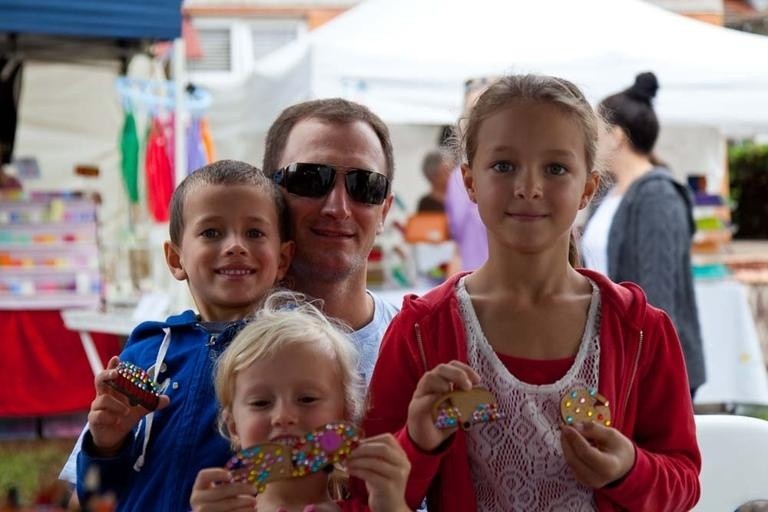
[273,161,393,208]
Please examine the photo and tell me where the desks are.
[0,287,130,444]
[673,275,767,415]
[60,305,148,378]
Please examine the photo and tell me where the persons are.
[68,157,295,512]
[56,93,403,512]
[566,72,708,412]
[391,75,498,284]
[334,76,703,511]
[179,284,416,511]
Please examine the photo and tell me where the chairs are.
[677,411,768,512]
